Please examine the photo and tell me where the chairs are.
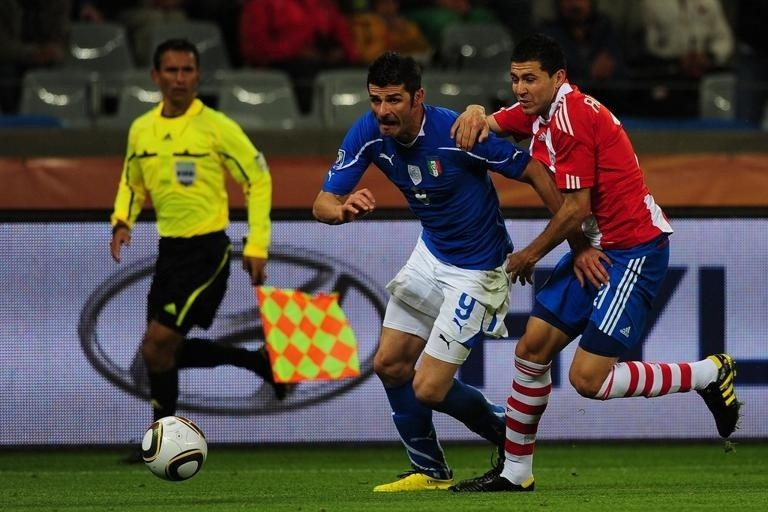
[50,21,134,95]
[312,70,370,121]
[117,73,163,120]
[217,72,298,120]
[20,72,100,121]
[419,70,491,111]
[150,22,234,94]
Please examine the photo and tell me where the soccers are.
[141,415,207,481]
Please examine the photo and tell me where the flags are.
[242,236,361,385]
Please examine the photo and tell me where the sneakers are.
[694,353,742,439]
[255,341,302,401]
[370,468,457,492]
[448,459,536,494]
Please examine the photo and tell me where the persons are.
[109,36,289,467]
[415,0,515,68]
[352,0,433,67]
[448,31,741,494]
[237,0,357,70]
[641,0,734,76]
[520,1,628,78]
[312,50,614,494]
